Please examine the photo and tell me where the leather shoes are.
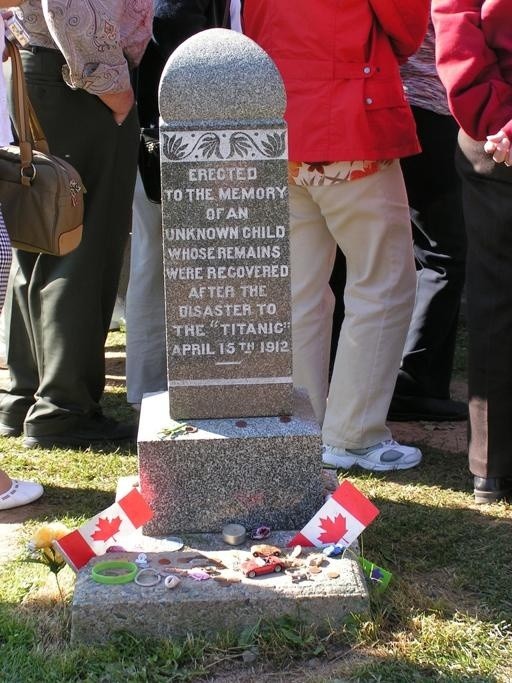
[0,478,44,510]
[473,474,507,505]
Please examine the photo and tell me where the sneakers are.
[321,427,423,471]
[386,393,471,422]
[22,411,137,449]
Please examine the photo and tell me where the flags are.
[281,481,383,556]
[51,489,156,572]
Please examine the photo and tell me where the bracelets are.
[92,560,137,583]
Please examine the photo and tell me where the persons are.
[241,1,511,504]
[0,469,44,511]
[0,0,241,444]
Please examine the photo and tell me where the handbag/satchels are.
[137,127,161,205]
[0,40,85,257]
